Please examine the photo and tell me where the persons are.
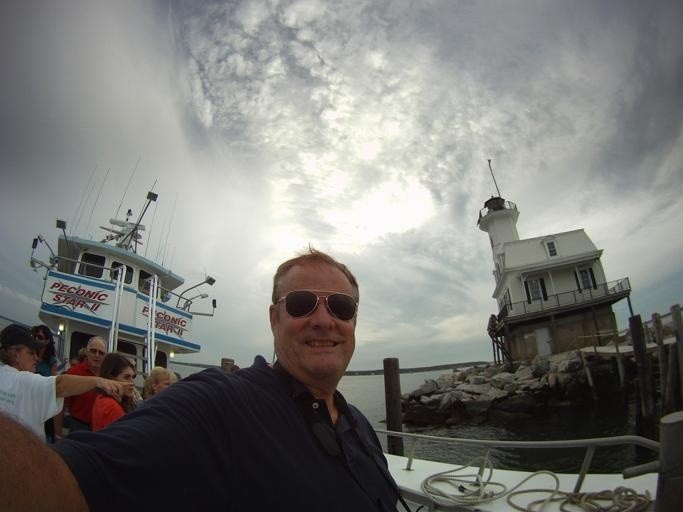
[53,335,108,444]
[92,354,136,432]
[30,325,57,444]
[0,250,401,511]
[0,323,135,443]
[143,366,177,401]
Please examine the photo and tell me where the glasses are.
[32,334,48,341]
[274,289,359,322]
[85,348,107,357]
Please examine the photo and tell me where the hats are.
[0,323,42,350]
